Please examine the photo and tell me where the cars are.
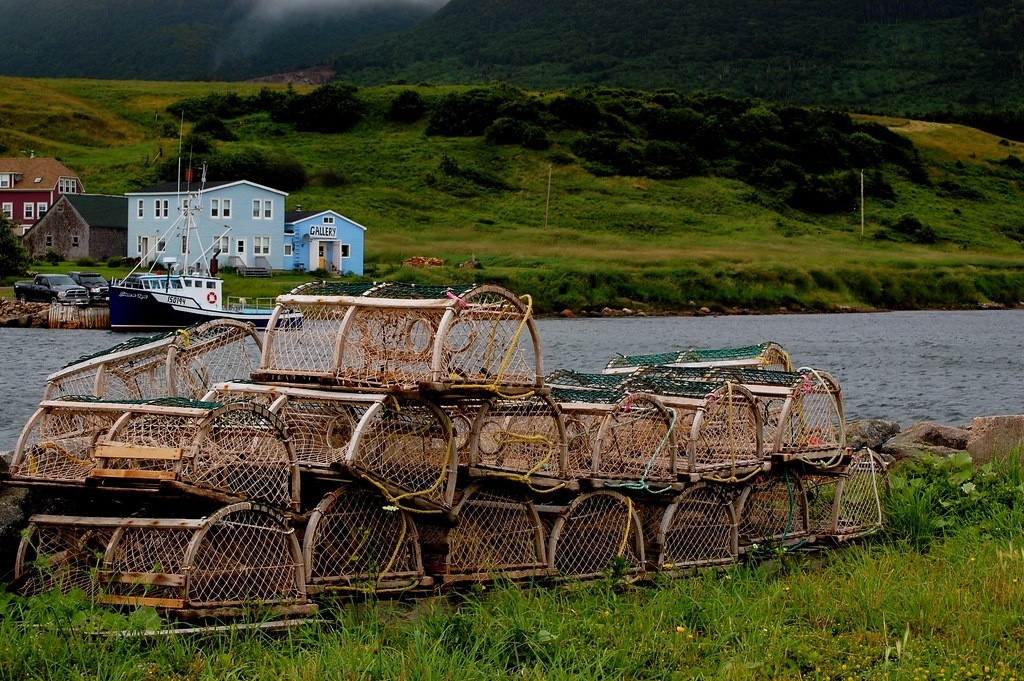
[67,271,110,307]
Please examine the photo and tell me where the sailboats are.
[110,109,305,333]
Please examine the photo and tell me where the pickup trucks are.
[13,273,91,310]
